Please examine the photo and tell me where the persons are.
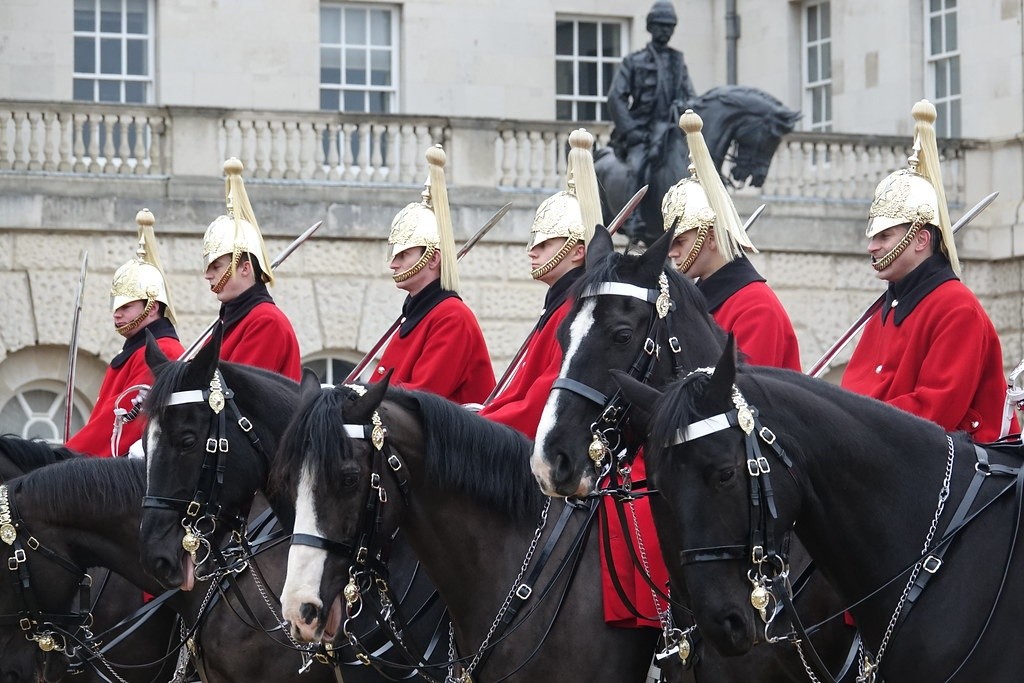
[366,201,496,409]
[599,179,802,628]
[475,191,587,444]
[202,217,300,381]
[606,1,700,232]
[63,260,189,603]
[840,171,1020,444]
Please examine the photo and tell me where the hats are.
[661,109,760,275]
[864,99,964,282]
[526,127,606,279]
[386,143,460,294]
[202,156,275,295]
[111,207,178,335]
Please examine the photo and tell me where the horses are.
[590,82,805,250]
[1,215,1024,683]
